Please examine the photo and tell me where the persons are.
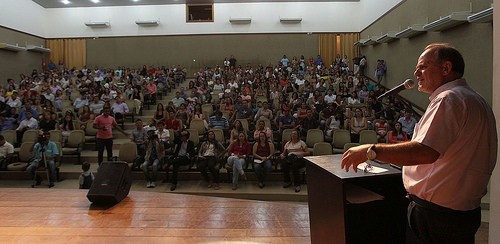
[0,54,417,192]
[340,42,498,244]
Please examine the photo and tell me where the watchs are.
[365,143,376,160]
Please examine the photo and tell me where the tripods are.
[31,143,51,188]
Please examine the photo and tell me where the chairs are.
[0,88,378,164]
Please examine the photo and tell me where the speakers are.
[87,162,132,205]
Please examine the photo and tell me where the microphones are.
[377,79,416,101]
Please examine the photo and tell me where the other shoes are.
[283,181,293,188]
[146,180,152,187]
[208,181,214,188]
[151,182,156,187]
[214,183,220,190]
[169,183,177,190]
[295,186,301,192]
[243,175,248,181]
[258,182,265,188]
[232,184,238,190]
[49,183,54,188]
[36,180,41,186]
